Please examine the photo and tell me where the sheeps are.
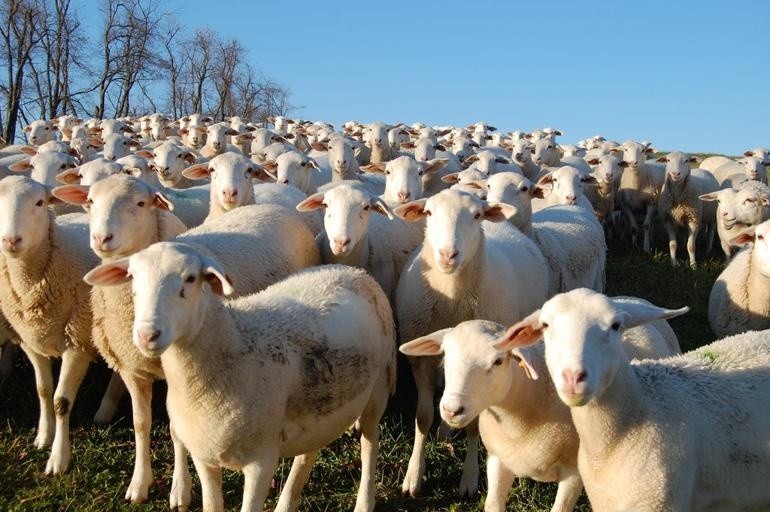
[1,112,770,512]
